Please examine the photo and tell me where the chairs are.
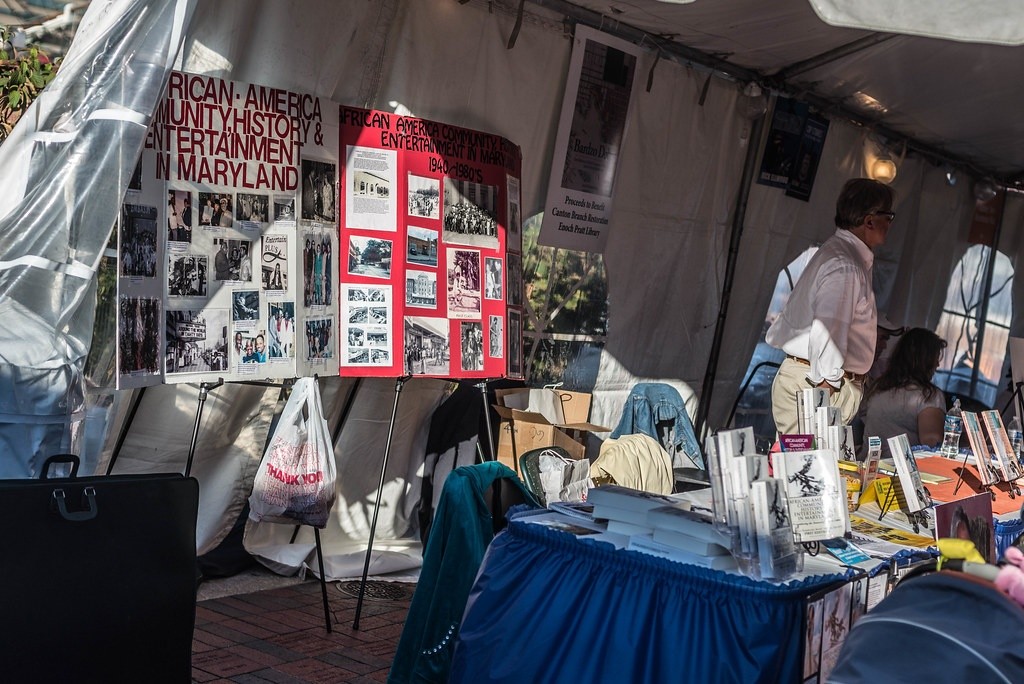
[387,460,548,684]
[612,383,711,494]
[519,445,573,508]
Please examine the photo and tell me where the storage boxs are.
[490,387,612,479]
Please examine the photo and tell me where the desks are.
[446,451,1024,684]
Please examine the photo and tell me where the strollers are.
[821,560,1024,684]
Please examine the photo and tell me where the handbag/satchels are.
[248,375,337,529]
[0,453,199,684]
[540,449,596,510]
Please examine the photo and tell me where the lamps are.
[736,82,767,121]
[872,153,897,183]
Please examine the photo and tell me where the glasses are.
[875,209,896,224]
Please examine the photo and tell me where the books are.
[548,387,1024,581]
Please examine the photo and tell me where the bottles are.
[941,399,964,457]
[1008,416,1022,460]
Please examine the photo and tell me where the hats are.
[876,312,906,336]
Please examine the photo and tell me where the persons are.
[1011,461,1021,476]
[851,316,906,454]
[763,178,893,443]
[851,580,865,629]
[916,489,926,508]
[859,331,947,462]
[922,486,932,507]
[805,606,818,674]
[117,169,522,373]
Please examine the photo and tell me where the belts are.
[786,354,866,382]
